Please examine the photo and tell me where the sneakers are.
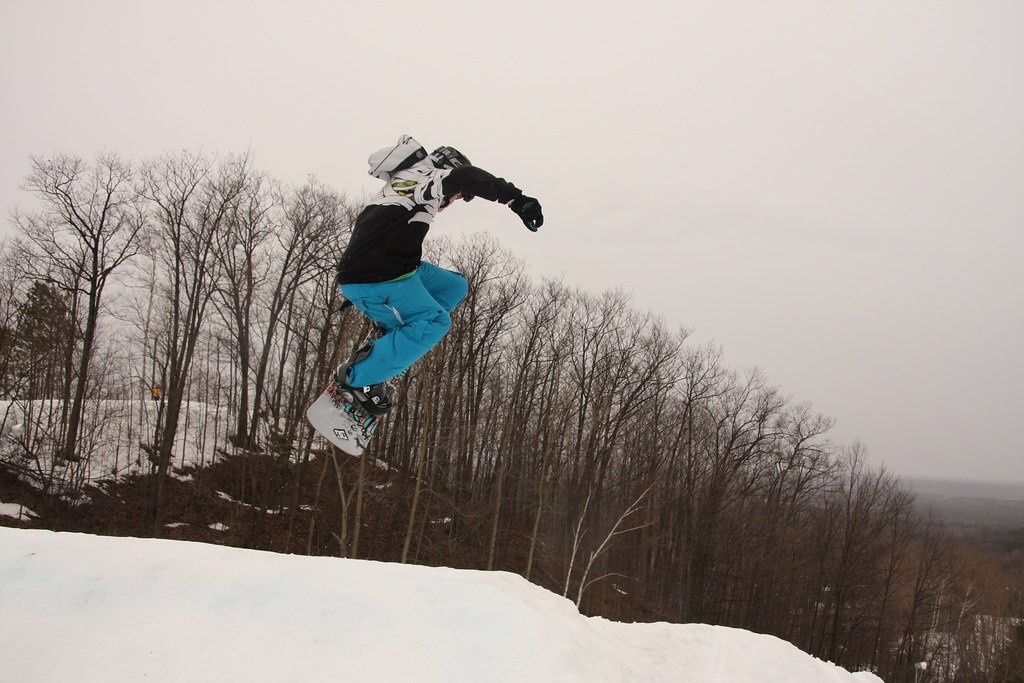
[367,322,387,345]
[334,363,392,416]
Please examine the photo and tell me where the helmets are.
[428,146,475,202]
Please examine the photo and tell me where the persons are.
[333,135,543,416]
[153,386,160,400]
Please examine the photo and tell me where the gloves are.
[510,195,543,232]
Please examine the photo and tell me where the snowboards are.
[305,321,411,458]
